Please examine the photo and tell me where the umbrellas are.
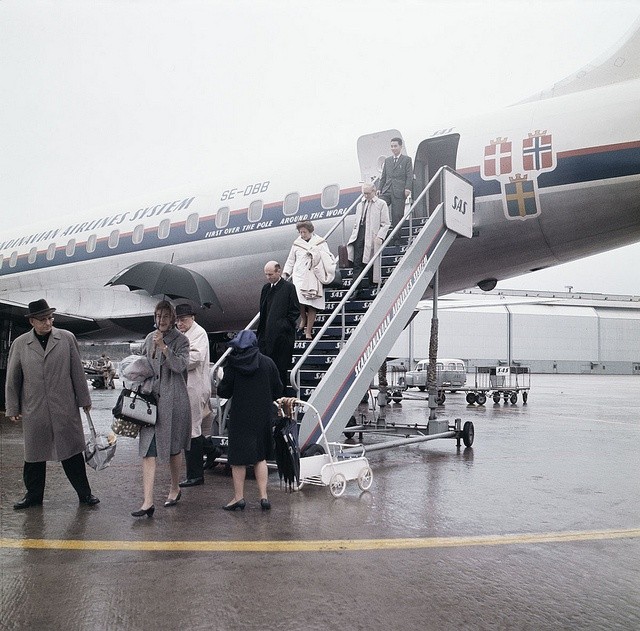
[274,397,300,494]
[104,252,225,360]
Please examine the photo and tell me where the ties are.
[394,158,397,162]
[361,199,369,225]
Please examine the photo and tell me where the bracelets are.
[160,345,167,350]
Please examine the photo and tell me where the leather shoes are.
[349,289,364,299]
[13,493,43,508]
[179,476,204,486]
[205,448,221,469]
[368,282,377,289]
[79,493,99,504]
[394,239,399,245]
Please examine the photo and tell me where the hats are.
[24,299,56,317]
[175,304,197,316]
[224,330,257,348]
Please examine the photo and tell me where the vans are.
[415,360,467,394]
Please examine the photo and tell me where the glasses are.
[32,317,55,322]
[363,191,373,195]
[175,317,193,323]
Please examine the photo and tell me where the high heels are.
[261,499,271,510]
[223,498,245,511]
[305,330,313,340]
[132,505,155,517]
[164,489,181,506]
[297,318,307,333]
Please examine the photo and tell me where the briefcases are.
[338,245,353,268]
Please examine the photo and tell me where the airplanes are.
[2,17,639,470]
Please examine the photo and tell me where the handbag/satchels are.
[85,431,116,471]
[113,385,157,427]
[111,417,141,438]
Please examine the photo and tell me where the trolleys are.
[276,398,374,500]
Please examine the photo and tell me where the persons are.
[283,221,335,337]
[346,183,391,300]
[217,330,283,511]
[100,354,105,365]
[104,356,116,389]
[376,137,413,246]
[174,304,213,487]
[4,299,99,509]
[256,261,301,386]
[130,301,192,517]
